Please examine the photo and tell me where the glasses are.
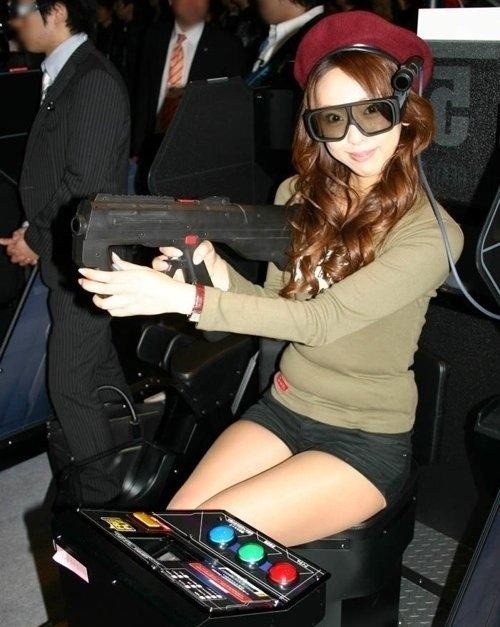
[304,90,405,141]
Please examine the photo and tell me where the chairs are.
[50,335,446,624]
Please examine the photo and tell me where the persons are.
[0,0,137,516]
[78,11,465,552]
[242,0,333,89]
[130,0,243,194]
[112,0,140,47]
[93,0,119,55]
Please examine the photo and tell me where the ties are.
[165,32,188,94]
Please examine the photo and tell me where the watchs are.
[187,283,206,324]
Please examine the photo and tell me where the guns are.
[71,193,305,298]
